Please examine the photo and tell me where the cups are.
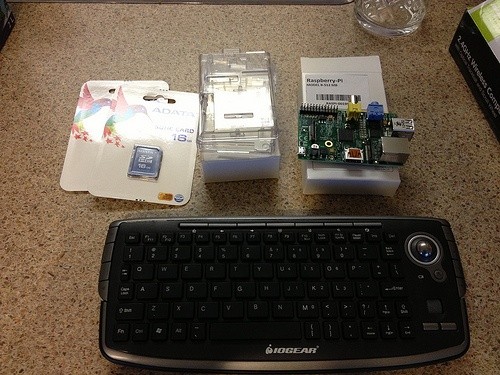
[354,0,426,39]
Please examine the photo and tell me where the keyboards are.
[97,213,472,375]
